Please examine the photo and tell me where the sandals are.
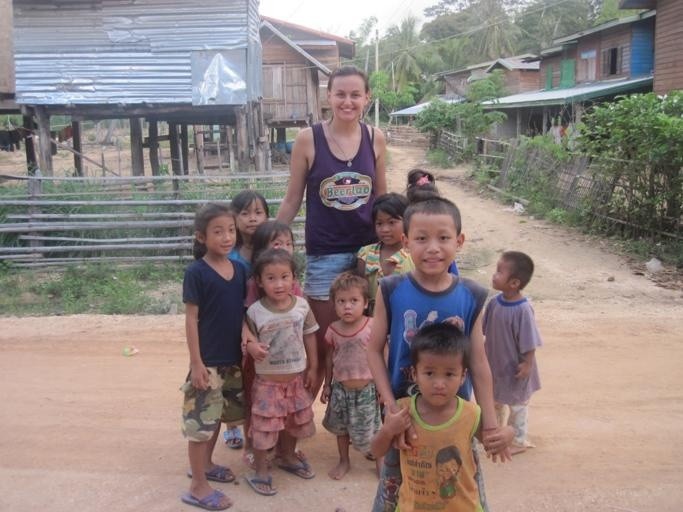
[244,451,270,469]
[294,448,307,460]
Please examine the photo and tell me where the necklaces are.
[326,122,361,167]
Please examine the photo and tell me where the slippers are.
[277,455,315,478]
[224,427,244,447]
[187,462,235,482]
[181,487,231,510]
[243,468,276,496]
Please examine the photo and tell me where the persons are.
[181,203,248,510]
[481,250,540,451]
[371,323,515,512]
[319,274,390,482]
[242,250,318,494]
[247,224,302,471]
[222,190,271,449]
[406,170,458,279]
[274,65,386,405]
[358,193,416,319]
[364,197,512,512]
